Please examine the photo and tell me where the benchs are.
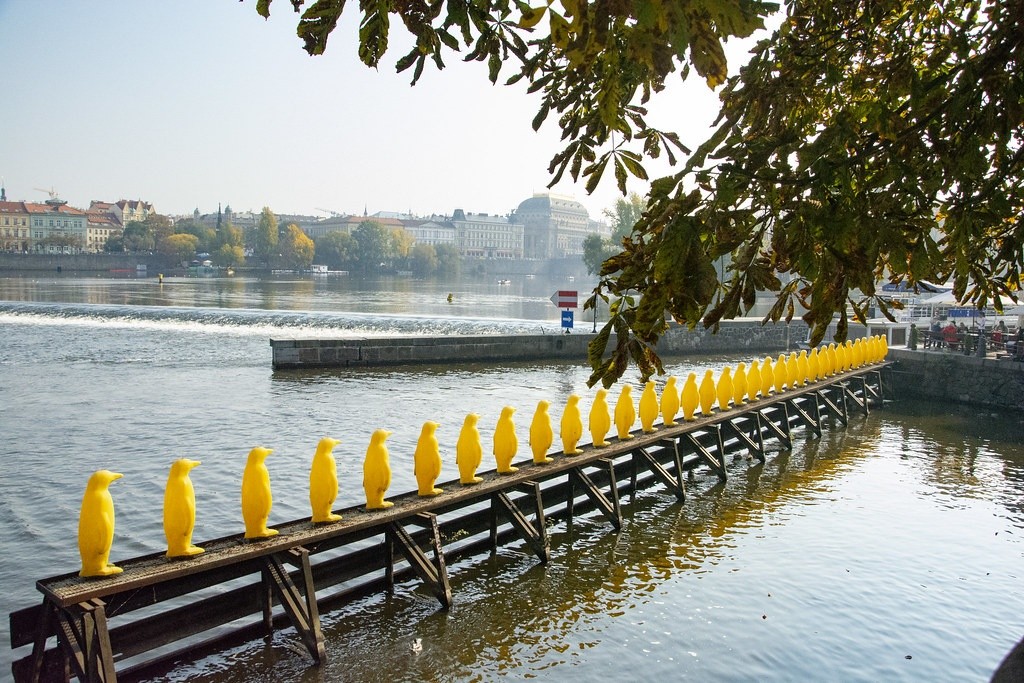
[921,328,1024,361]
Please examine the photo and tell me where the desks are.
[986,333,1017,341]
[958,333,980,351]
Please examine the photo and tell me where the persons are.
[926,312,1010,352]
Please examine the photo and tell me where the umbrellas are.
[924,280,1024,352]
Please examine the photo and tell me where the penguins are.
[78,332,889,578]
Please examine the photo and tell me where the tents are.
[882,274,954,302]
